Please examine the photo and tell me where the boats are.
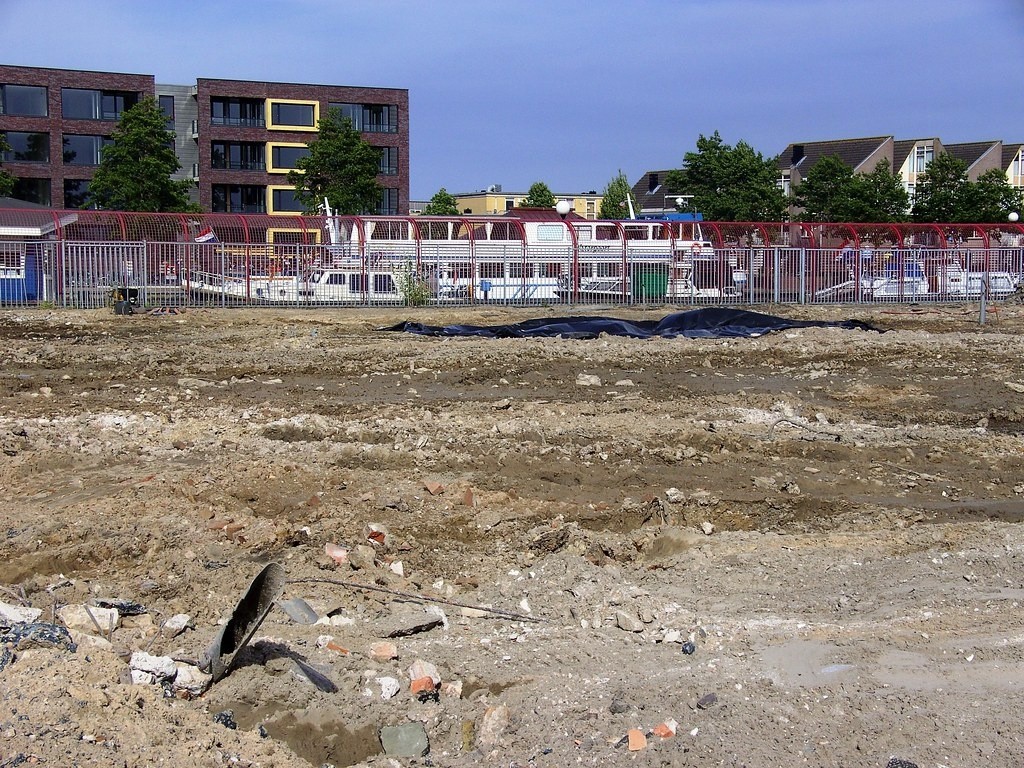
[551,259,744,306]
[857,272,1019,301]
[179,267,472,307]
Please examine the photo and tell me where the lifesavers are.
[691,243,702,255]
[429,264,459,291]
